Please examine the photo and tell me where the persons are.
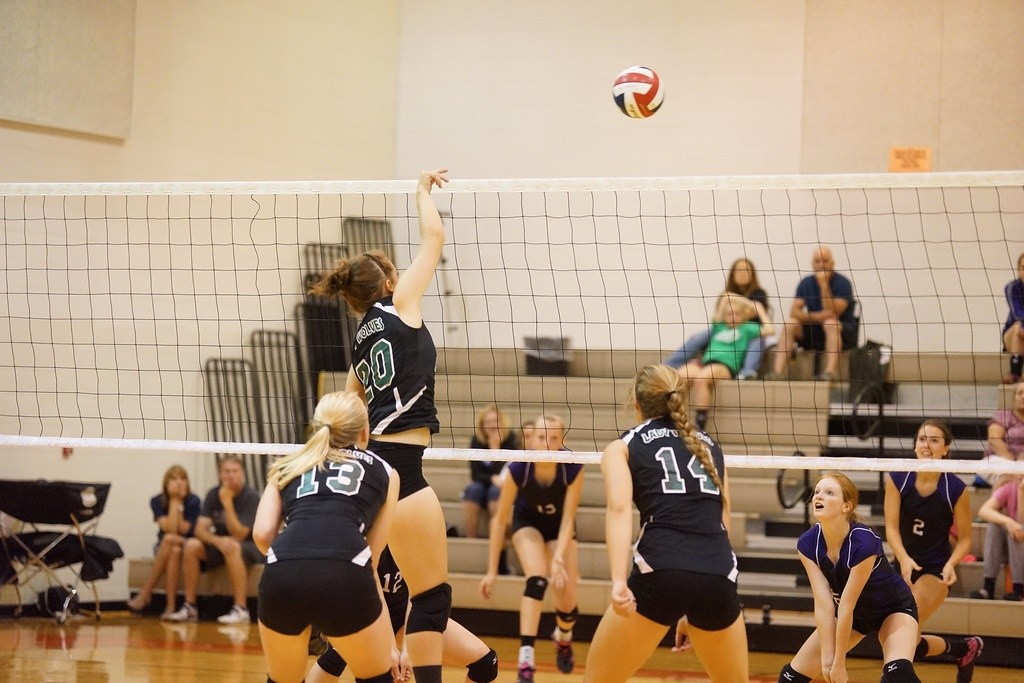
[778,472,922,683]
[663,257,775,431]
[304,169,498,683]
[761,247,855,381]
[969,254,1024,601]
[161,455,268,625]
[127,464,200,620]
[479,414,579,682]
[458,404,535,538]
[584,363,748,683]
[884,420,983,682]
[252,391,400,683]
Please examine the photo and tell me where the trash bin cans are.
[524,337,570,375]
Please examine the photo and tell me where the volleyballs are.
[611,65,666,120]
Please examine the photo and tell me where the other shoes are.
[818,373,837,382]
[127,594,148,611]
[161,609,173,620]
[764,370,784,382]
[553,630,573,674]
[737,370,756,380]
[1003,591,1023,601]
[517,663,536,683]
[970,587,993,601]
[170,602,198,621]
[217,605,250,624]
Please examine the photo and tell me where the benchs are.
[129,349,1024,644]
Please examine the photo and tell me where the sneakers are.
[956,637,983,682]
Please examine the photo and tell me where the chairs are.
[0,478,112,624]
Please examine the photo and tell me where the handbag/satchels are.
[849,341,893,404]
